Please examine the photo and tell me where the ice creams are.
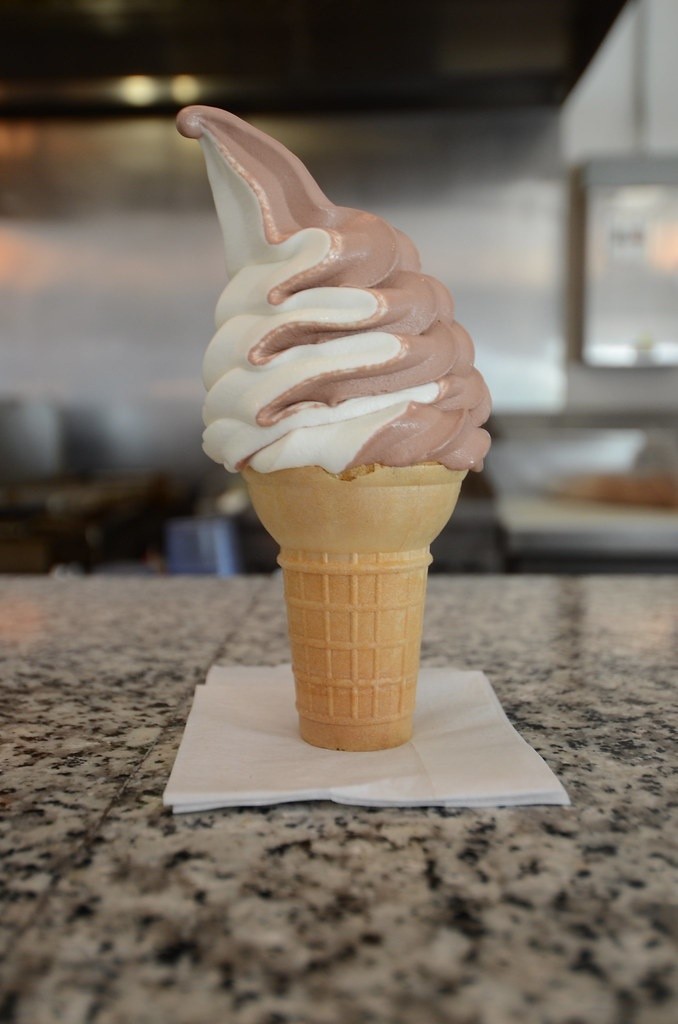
[175,105,497,752]
